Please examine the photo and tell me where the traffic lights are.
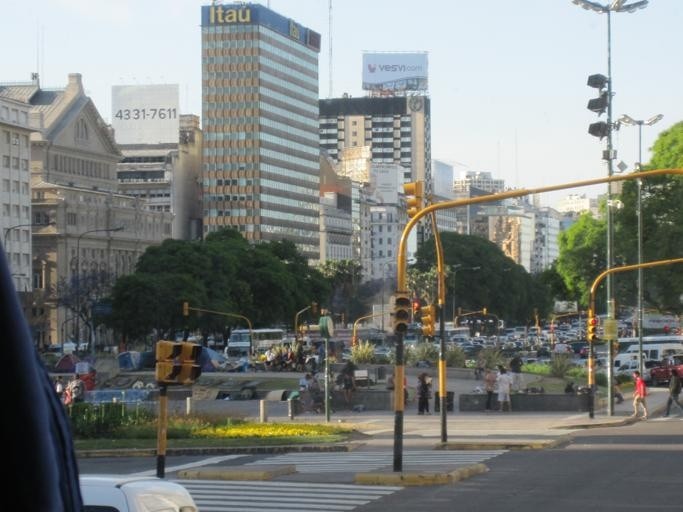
[312,299,319,317]
[389,290,412,334]
[416,301,433,339]
[178,341,203,383]
[155,340,182,386]
[399,180,418,221]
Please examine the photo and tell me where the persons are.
[52,367,97,404]
[613,381,626,403]
[630,370,649,419]
[217,335,525,415]
[661,368,682,418]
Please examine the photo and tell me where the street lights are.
[617,113,664,415]
[451,264,484,322]
[72,225,129,356]
[2,221,57,252]
[565,0,654,419]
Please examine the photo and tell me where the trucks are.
[224,329,283,359]
[48,342,86,358]
[612,300,682,387]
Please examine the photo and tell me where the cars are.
[78,473,198,511]
[332,316,615,361]
